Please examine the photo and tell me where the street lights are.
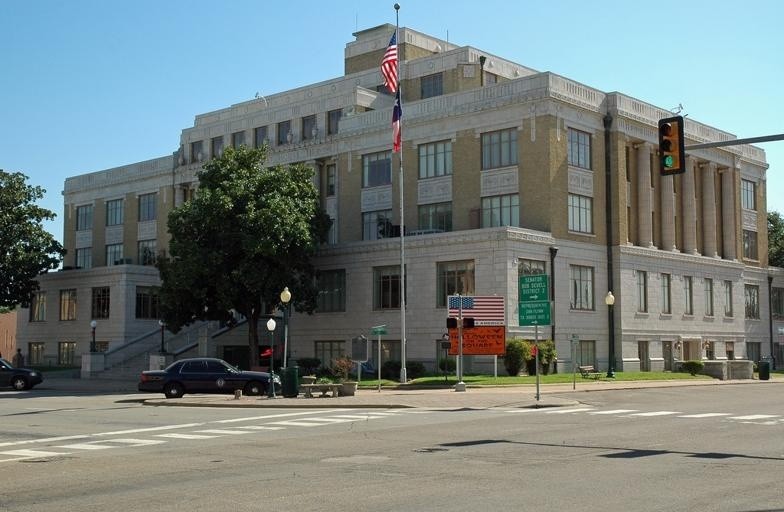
[280,286,293,367]
[158,318,168,356]
[267,318,277,397]
[605,289,617,379]
[90,320,99,352]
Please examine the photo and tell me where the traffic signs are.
[517,300,552,327]
[517,275,549,300]
[371,324,388,335]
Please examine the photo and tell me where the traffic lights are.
[462,317,476,329]
[446,317,460,330]
[659,116,685,178]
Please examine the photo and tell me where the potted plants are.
[504,338,558,376]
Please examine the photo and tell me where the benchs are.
[578,365,602,379]
[299,382,343,399]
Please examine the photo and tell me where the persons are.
[11,349,24,368]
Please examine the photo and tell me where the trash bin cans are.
[280,366,299,398]
[758,361,770,380]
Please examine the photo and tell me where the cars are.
[138,358,282,398]
[0,358,45,390]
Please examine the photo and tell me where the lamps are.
[675,338,683,351]
[702,340,711,351]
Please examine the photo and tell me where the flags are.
[391,83,402,150]
[380,29,398,93]
